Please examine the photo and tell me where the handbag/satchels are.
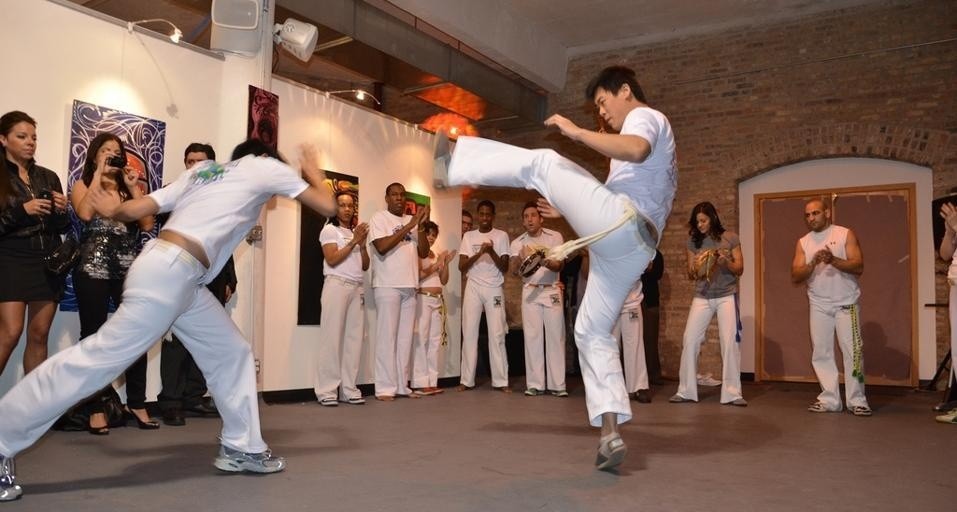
[37,187,81,285]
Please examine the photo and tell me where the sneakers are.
[847,403,870,417]
[808,403,829,412]
[0,456,22,502]
[213,445,286,474]
[525,388,546,396]
[935,410,955,422]
[697,372,723,388]
[322,396,340,406]
[342,397,367,405]
[547,389,570,398]
[593,431,627,471]
[431,129,452,192]
[415,388,436,395]
[431,387,445,394]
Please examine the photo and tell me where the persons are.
[936,202,956,424]
[454,200,513,394]
[367,182,432,400]
[411,221,457,396]
[431,65,677,471]
[641,248,664,385]
[461,208,474,239]
[791,200,873,417]
[510,201,570,397]
[68,131,160,435]
[1,139,336,502]
[0,111,67,380]
[314,190,371,406]
[670,203,749,407]
[563,255,590,382]
[157,143,234,424]
[611,279,651,403]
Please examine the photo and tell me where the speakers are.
[209,1,265,56]
[281,19,317,62]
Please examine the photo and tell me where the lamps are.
[127,18,184,43]
[326,90,380,105]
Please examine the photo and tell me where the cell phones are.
[36,188,50,199]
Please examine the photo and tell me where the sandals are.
[497,387,513,394]
[377,395,394,402]
[397,393,422,399]
[457,385,475,392]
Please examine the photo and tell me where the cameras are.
[108,157,126,169]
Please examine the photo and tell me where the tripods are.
[928,349,955,410]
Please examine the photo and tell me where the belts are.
[416,291,445,297]
[325,275,365,288]
[522,282,558,289]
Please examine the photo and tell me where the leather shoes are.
[730,396,749,406]
[636,390,654,404]
[669,393,697,403]
[161,410,186,427]
[184,404,220,418]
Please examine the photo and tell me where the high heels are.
[123,401,158,429]
[87,409,110,435]
